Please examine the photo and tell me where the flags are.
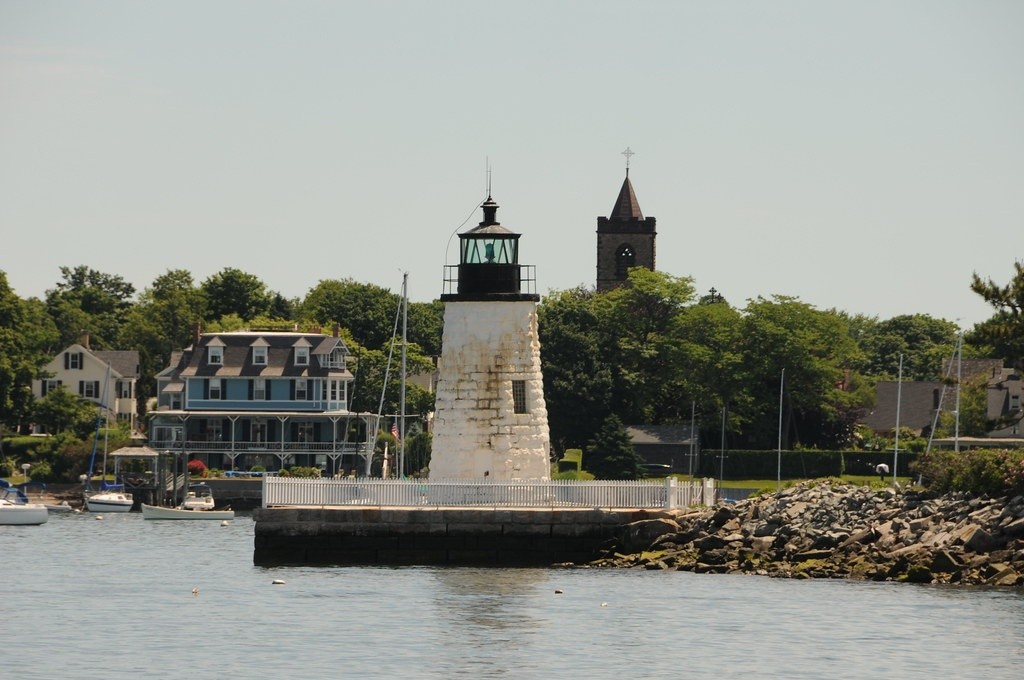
[391,423,400,442]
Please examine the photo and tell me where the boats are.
[0,477,49,525]
[141,484,235,523]
[43,499,71,511]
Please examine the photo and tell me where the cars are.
[634,461,673,479]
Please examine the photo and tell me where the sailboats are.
[83,360,135,518]
